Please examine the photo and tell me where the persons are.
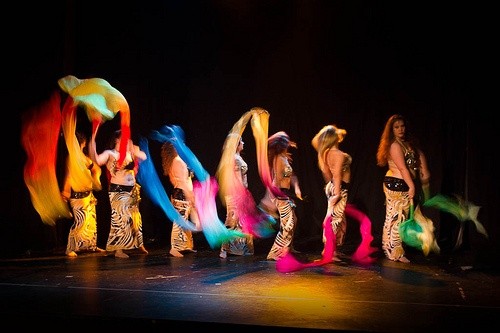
[376,116,430,264]
[219,133,255,258]
[311,125,352,262]
[61,135,105,256]
[267,131,305,262]
[162,139,200,257]
[92,130,149,257]
